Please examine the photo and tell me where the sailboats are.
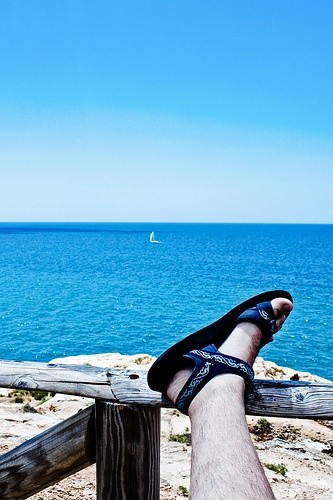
[149,232,161,243]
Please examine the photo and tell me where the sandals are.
[147,290,293,416]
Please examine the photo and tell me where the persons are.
[147,290,293,500]
